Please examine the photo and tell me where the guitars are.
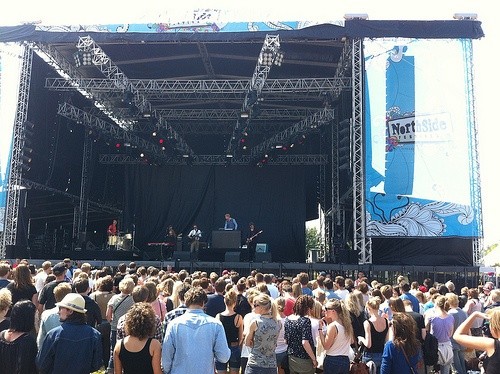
[189,231,204,243]
[246,230,263,244]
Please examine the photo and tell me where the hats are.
[53,263,67,274]
[395,280,410,289]
[54,293,87,314]
[419,285,426,293]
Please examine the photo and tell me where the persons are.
[74,279,102,329]
[105,219,118,251]
[37,263,70,311]
[163,286,190,340]
[166,224,177,260]
[224,214,238,231]
[188,225,202,262]
[0,259,500,374]
[245,222,261,245]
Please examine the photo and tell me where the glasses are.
[324,306,334,311]
[254,304,259,308]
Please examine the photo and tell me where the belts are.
[231,341,239,346]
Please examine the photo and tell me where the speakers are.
[255,252,272,264]
[172,251,191,261]
[225,252,244,262]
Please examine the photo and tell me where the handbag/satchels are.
[349,359,368,374]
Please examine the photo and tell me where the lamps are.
[18,164,32,172]
[22,130,34,138]
[22,139,33,146]
[262,51,273,66]
[274,50,288,67]
[20,155,32,163]
[21,120,35,129]
[71,50,93,66]
[21,148,33,154]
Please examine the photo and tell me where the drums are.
[118,238,131,250]
[108,235,120,245]
[121,233,132,240]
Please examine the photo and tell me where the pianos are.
[146,242,176,260]
